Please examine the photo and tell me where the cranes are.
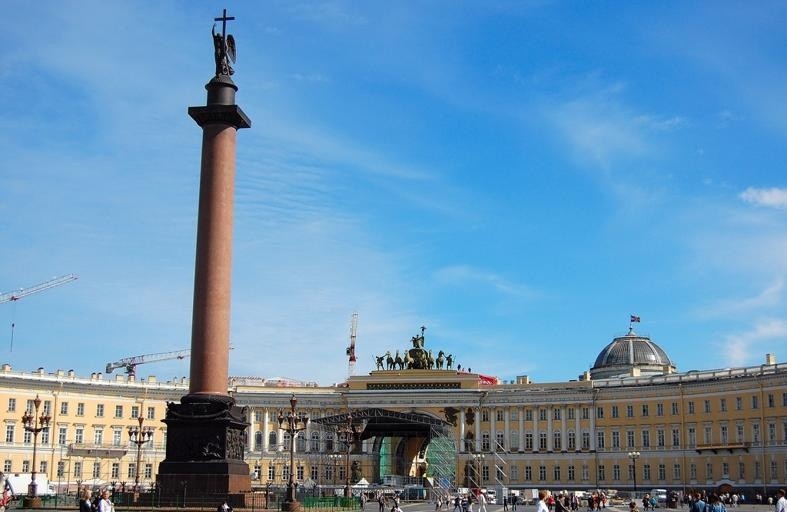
[105,346,236,377]
[0,272,79,353]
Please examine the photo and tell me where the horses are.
[385,348,445,371]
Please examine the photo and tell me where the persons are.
[377,492,404,512]
[445,354,454,371]
[535,488,607,512]
[478,490,488,512]
[375,354,387,371]
[504,493,518,511]
[78,488,115,512]
[435,492,474,512]
[359,492,367,511]
[678,488,745,512]
[629,493,656,512]
[756,487,787,512]
[410,334,423,349]
[216,501,234,512]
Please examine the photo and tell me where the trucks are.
[384,474,403,486]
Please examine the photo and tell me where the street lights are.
[22,395,51,484]
[336,412,362,498]
[628,451,640,498]
[128,413,153,485]
[471,452,485,487]
[277,393,308,503]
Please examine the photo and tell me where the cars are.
[395,483,428,500]
